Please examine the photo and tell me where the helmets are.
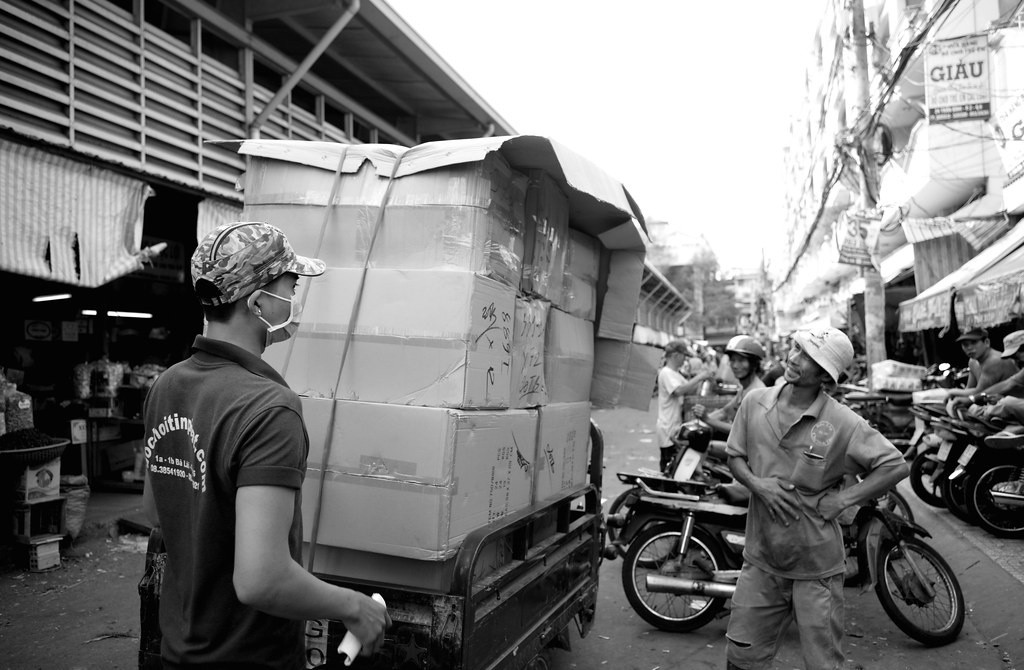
[724,334,764,360]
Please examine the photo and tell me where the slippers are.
[984,430,1024,450]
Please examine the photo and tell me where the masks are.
[247,289,303,347]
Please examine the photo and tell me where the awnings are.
[896,217,1024,334]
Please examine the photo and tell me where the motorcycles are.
[119,410,616,669]
[608,368,1024,650]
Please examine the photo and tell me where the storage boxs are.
[242,151,665,593]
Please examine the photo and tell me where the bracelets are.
[967,394,976,406]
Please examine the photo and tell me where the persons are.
[723,323,911,670]
[693,335,769,483]
[658,340,715,471]
[955,330,1023,451]
[138,221,399,670]
[941,325,1023,417]
[679,339,729,388]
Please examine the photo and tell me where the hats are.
[191,221,328,306]
[665,341,694,358]
[791,326,855,393]
[955,327,989,343]
[1001,329,1024,360]
